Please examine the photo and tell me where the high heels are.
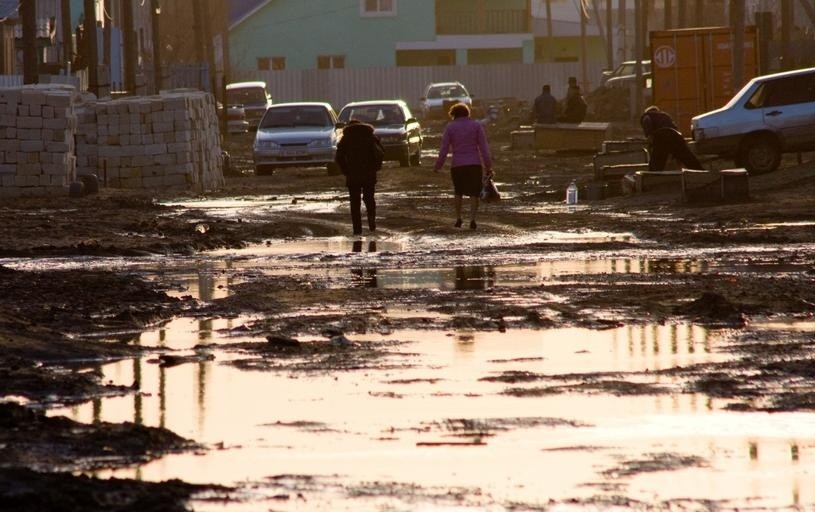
[454,218,477,229]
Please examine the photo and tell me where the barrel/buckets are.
[566,181,578,205]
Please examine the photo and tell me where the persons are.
[640,105,705,171]
[568,76,577,87]
[433,103,496,229]
[559,84,587,123]
[350,240,378,289]
[335,120,384,236]
[457,263,494,290]
[534,84,556,124]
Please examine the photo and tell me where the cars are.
[690,67,815,176]
[226,81,424,176]
[419,82,475,117]
[601,59,652,96]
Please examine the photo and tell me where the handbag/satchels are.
[480,176,500,202]
